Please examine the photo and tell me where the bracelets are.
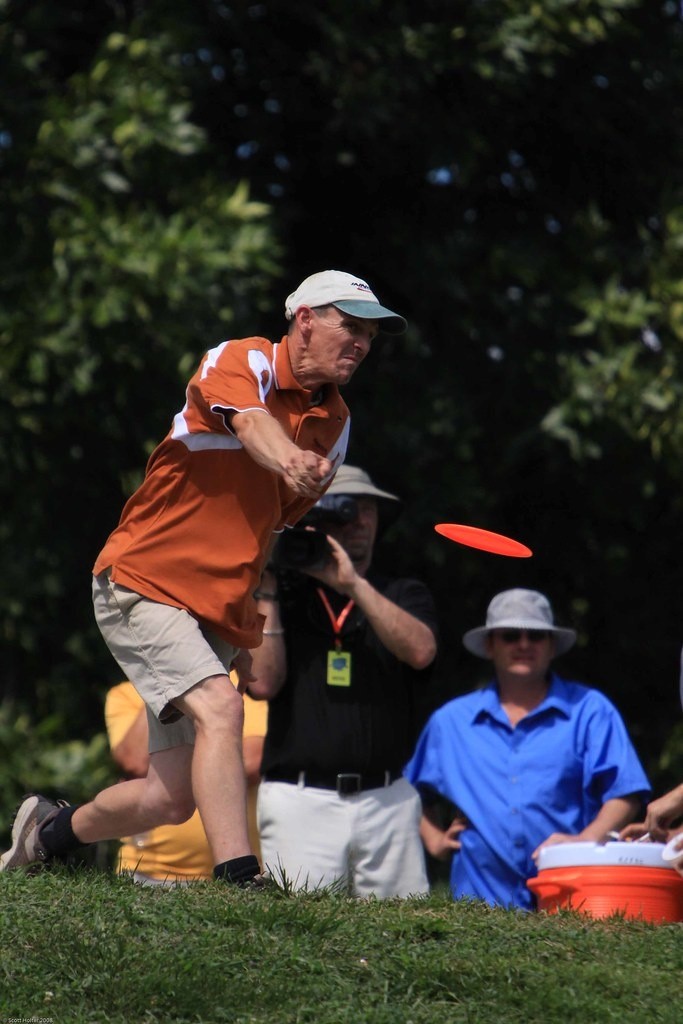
[253,592,283,602]
[263,628,285,636]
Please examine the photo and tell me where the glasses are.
[503,630,546,643]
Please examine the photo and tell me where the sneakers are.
[0,794,71,872]
[244,871,282,893]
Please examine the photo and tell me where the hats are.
[462,588,577,658]
[285,270,408,336]
[323,464,399,501]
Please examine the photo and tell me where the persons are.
[402,587,651,916]
[232,466,434,902]
[619,781,683,850]
[0,267,408,902]
[106,667,269,886]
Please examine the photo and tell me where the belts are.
[266,769,403,795]
